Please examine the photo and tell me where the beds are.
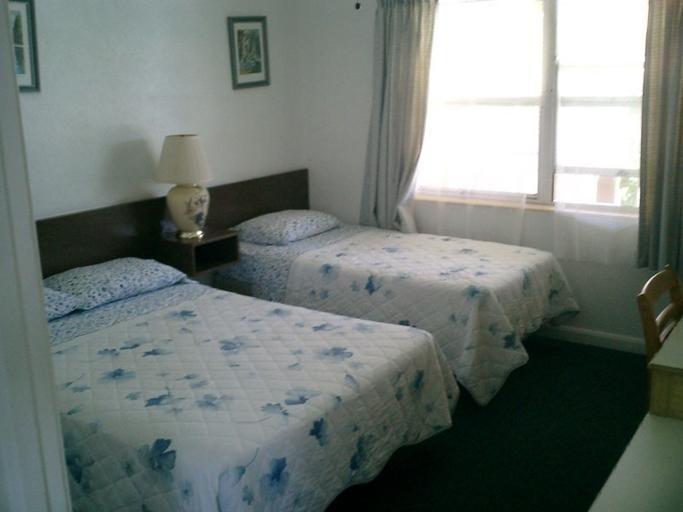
[42,275,432,512]
[226,222,552,382]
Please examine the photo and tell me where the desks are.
[586,319,683,512]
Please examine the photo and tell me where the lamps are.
[153,133,215,241]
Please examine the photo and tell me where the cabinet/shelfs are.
[163,226,240,277]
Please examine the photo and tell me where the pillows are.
[41,257,186,322]
[231,209,345,246]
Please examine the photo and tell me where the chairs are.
[637,263,682,356]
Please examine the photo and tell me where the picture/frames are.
[7,0,39,92]
[226,16,269,89]
